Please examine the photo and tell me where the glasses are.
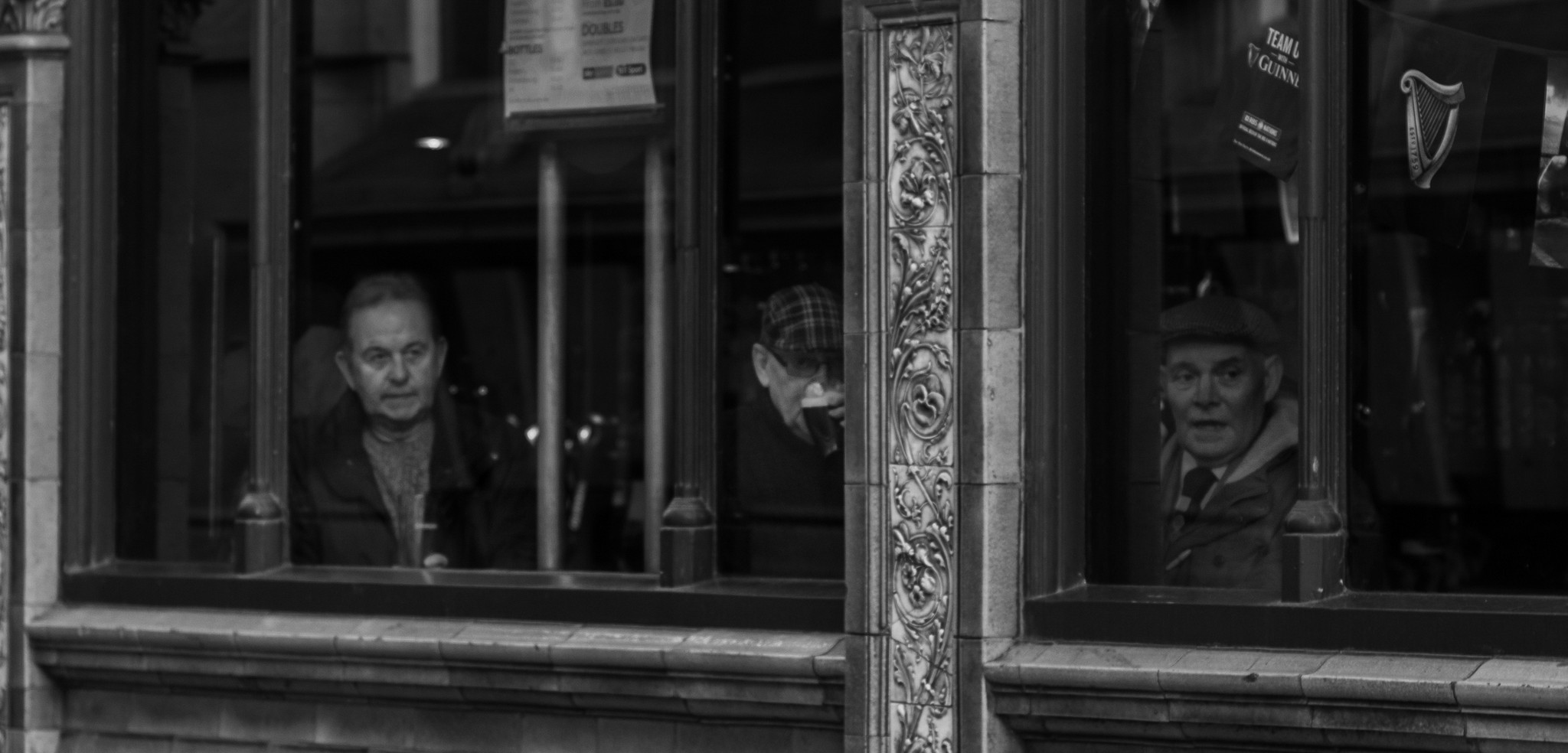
[767,348,844,387]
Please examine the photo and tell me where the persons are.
[1157,297,1382,591]
[289,272,537,572]
[715,286,846,580]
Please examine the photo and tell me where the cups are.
[801,391,844,457]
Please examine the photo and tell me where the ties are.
[1180,467,1219,515]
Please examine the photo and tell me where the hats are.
[1159,295,1277,354]
[762,283,845,360]
